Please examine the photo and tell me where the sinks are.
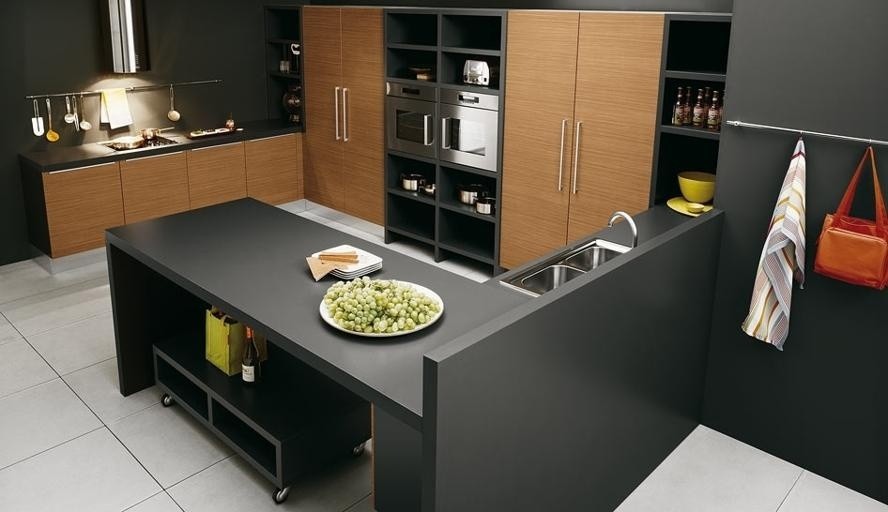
[499,258,587,298]
[563,238,633,272]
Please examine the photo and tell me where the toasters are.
[462,58,498,88]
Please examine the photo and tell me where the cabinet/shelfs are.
[187,141,248,210]
[264,4,306,131]
[42,162,125,259]
[651,13,732,215]
[377,7,507,95]
[384,149,499,275]
[120,150,190,225]
[244,133,303,206]
[500,11,665,273]
[301,3,385,227]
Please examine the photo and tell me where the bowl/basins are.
[407,63,435,74]
[687,203,705,213]
[678,172,717,203]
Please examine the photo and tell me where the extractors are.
[98,0,153,76]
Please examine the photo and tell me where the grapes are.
[325,276,439,332]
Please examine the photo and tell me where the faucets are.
[607,211,638,250]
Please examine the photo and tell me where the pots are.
[401,172,436,196]
[110,125,176,149]
[476,197,496,215]
[458,184,486,204]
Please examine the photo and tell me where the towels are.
[100,88,134,130]
[741,138,807,352]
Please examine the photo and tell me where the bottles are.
[670,86,726,129]
[241,325,261,384]
[280,60,290,72]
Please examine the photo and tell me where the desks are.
[612,424,888,511]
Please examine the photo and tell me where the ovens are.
[386,77,500,173]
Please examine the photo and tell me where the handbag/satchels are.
[813,146,888,291]
[205,305,269,376]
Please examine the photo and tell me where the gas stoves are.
[104,134,177,153]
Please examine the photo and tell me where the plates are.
[321,279,445,338]
[311,243,383,282]
[191,119,244,137]
[666,196,713,217]
